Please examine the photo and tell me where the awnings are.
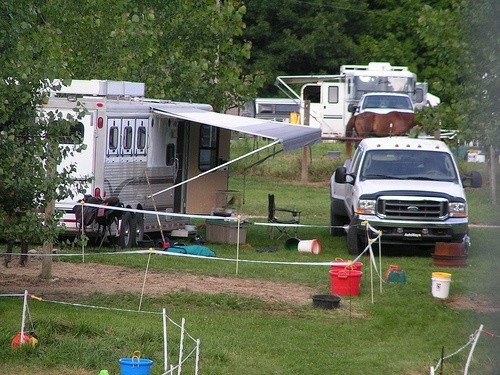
[274,75,346,137]
[146,106,322,199]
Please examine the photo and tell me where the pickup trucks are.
[329,137,482,256]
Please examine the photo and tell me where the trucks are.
[254,62,441,140]
[4,76,232,249]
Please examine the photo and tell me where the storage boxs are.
[205,219,249,245]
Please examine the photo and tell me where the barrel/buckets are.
[329,270,362,296]
[431,272,451,297]
[298,239,320,254]
[331,262,362,271]
[119,358,153,375]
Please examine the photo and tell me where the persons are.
[416,158,446,175]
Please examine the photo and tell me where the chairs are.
[268,193,301,240]
